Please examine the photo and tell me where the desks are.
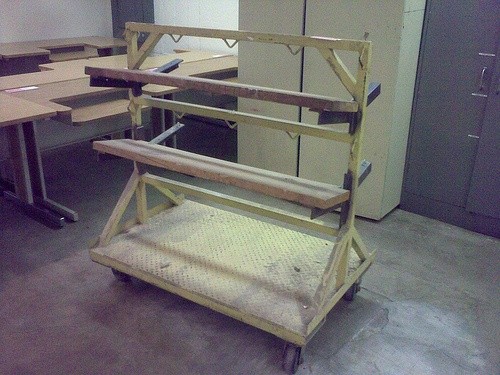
[0,35,238,233]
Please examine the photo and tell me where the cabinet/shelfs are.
[88,21,379,375]
[237,0,427,223]
